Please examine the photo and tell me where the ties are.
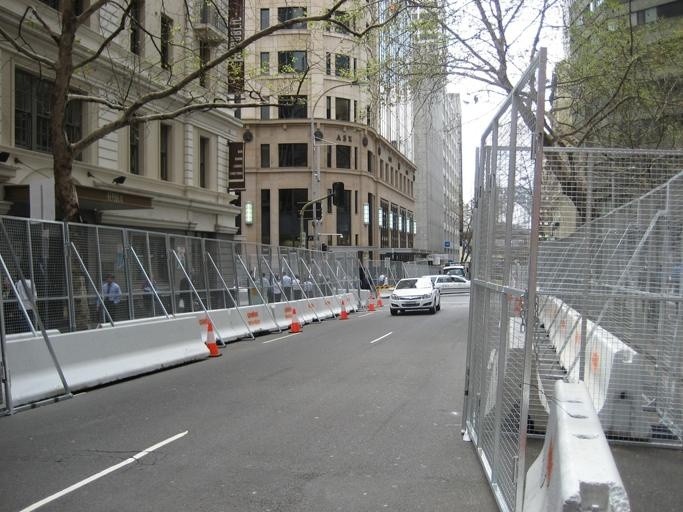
[105,283,110,301]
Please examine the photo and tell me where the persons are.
[16,274,38,332]
[378,271,384,282]
[179,271,195,312]
[96,274,121,323]
[142,273,157,318]
[247,269,333,306]
[2,276,19,334]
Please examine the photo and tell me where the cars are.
[425,275,470,290]
[389,277,440,314]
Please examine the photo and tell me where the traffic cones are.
[366,294,376,311]
[376,292,384,307]
[338,300,349,320]
[287,307,304,333]
[202,320,222,358]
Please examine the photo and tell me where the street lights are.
[310,80,368,304]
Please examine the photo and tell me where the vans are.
[442,265,466,279]
